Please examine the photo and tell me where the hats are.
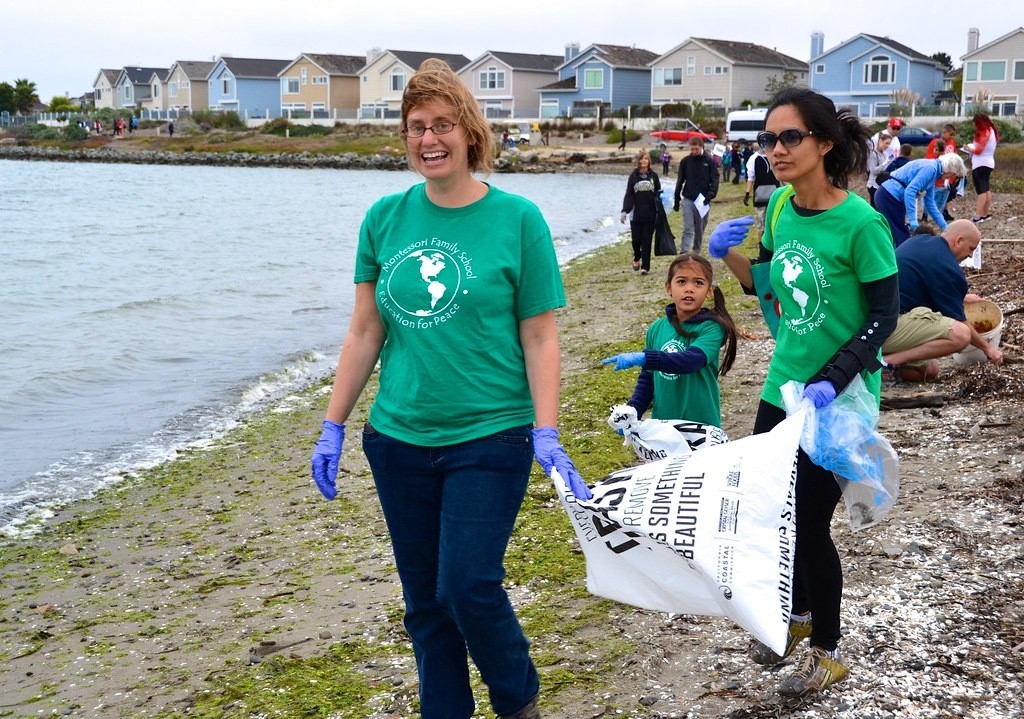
[889,117,903,128]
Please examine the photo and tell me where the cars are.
[896,128,940,145]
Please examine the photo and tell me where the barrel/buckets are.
[953,300,1003,365]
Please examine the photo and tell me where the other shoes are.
[943,213,954,221]
[501,695,542,719]
[881,365,898,387]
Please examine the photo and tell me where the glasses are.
[890,126,901,131]
[757,128,821,152]
[401,120,465,138]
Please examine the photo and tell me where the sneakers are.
[749,617,812,666]
[984,213,992,221]
[970,216,983,224]
[777,647,850,697]
[641,269,648,275]
[633,261,640,271]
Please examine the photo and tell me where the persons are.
[867,132,894,210]
[708,83,899,697]
[132,116,138,136]
[621,149,661,275]
[169,121,174,138]
[94,120,100,133]
[743,147,788,250]
[874,153,967,249]
[600,252,738,431]
[501,129,509,150]
[113,118,127,137]
[963,111,999,223]
[866,118,901,157]
[315,63,593,719]
[662,150,672,175]
[673,137,719,255]
[128,116,133,135]
[881,219,1002,385]
[923,124,958,222]
[884,144,913,172]
[714,138,754,184]
[912,225,937,236]
[618,125,627,152]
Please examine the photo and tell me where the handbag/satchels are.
[754,185,776,203]
[749,185,794,341]
[875,170,907,189]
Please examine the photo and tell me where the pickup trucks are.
[501,128,530,144]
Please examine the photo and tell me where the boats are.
[649,130,719,142]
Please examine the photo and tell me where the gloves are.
[703,195,712,205]
[311,419,346,501]
[708,215,755,258]
[531,426,591,502]
[744,192,749,206]
[616,428,634,436]
[673,201,680,212]
[802,381,836,409]
[620,211,626,224]
[602,351,646,371]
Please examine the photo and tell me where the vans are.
[725,109,769,142]
